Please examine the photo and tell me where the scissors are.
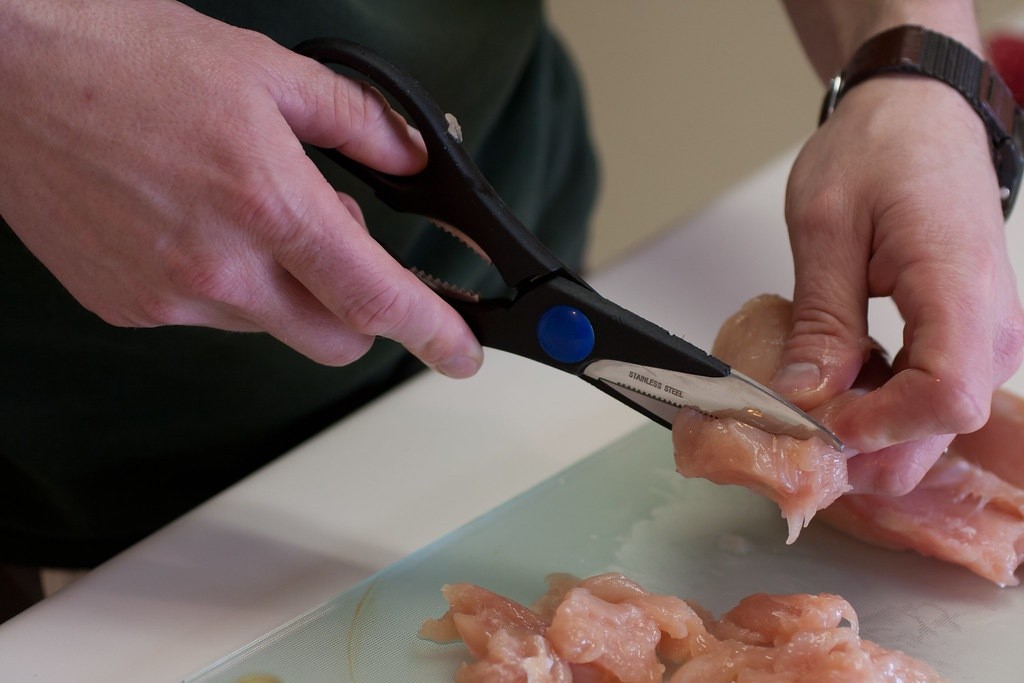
[262,36,847,455]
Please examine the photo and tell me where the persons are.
[1,1,1022,571]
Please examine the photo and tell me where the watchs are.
[818,25,1022,225]
